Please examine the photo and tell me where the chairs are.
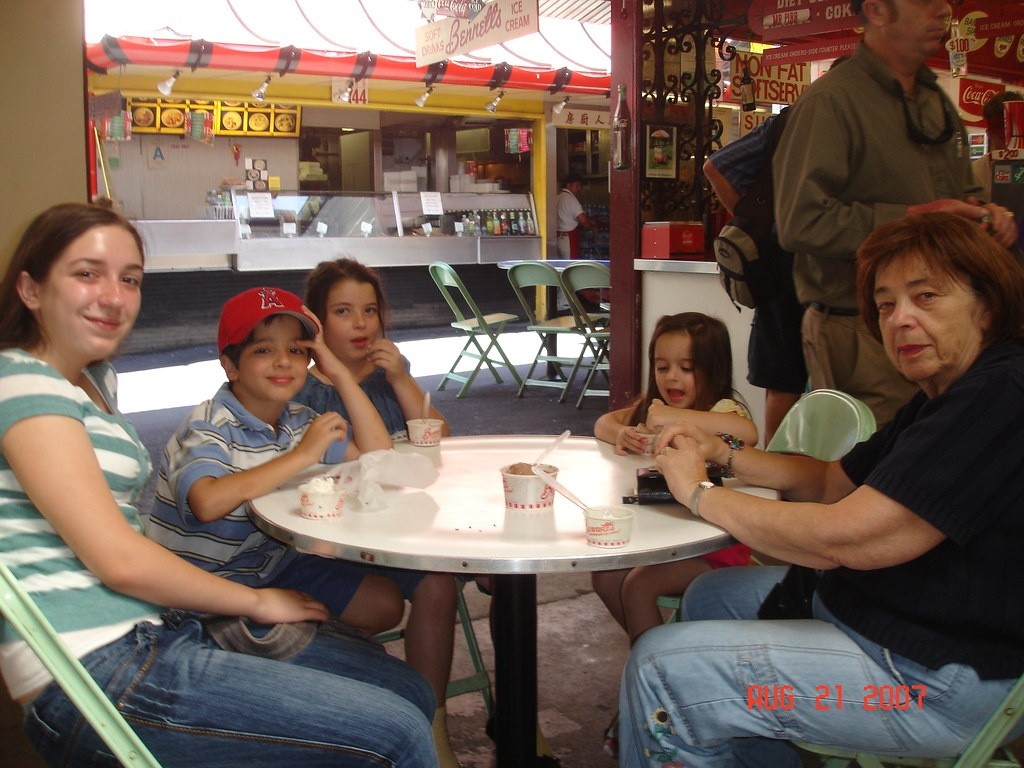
[506,262,609,403]
[369,579,493,720]
[655,389,877,624]
[561,262,610,408]
[428,262,526,398]
[792,672,1024,768]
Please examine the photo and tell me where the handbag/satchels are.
[713,208,785,309]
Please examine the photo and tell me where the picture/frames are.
[640,120,681,184]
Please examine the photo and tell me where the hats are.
[218,287,320,355]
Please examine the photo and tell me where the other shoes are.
[486,717,564,767]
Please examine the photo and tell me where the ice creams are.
[636,423,663,434]
[300,477,341,492]
[504,462,553,475]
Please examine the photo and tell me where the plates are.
[248,159,266,190]
[133,97,296,133]
[492,190,510,193]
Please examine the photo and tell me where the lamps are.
[484,93,505,114]
[339,82,354,102]
[156,70,182,96]
[251,76,271,102]
[552,97,569,114]
[414,87,434,108]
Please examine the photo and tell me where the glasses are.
[896,78,956,145]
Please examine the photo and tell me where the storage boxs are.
[640,222,705,262]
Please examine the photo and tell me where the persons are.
[291,257,497,732]
[1,201,440,768]
[555,174,600,314]
[615,211,1024,768]
[773,0,1017,432]
[703,54,853,449]
[143,286,406,632]
[594,311,759,645]
[970,87,1024,204]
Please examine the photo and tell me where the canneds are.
[467,161,476,175]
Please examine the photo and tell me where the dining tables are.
[498,259,610,382]
[248,434,781,768]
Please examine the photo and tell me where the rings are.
[662,448,671,455]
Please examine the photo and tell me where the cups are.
[1002,100,1024,150]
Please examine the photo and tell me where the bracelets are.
[708,431,747,478]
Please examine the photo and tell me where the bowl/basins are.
[636,432,658,456]
[499,463,559,511]
[405,418,445,446]
[297,483,345,520]
[584,506,636,549]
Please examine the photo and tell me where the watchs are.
[688,482,716,518]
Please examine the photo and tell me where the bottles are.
[612,84,631,169]
[740,63,757,112]
[949,19,968,79]
[461,211,535,236]
